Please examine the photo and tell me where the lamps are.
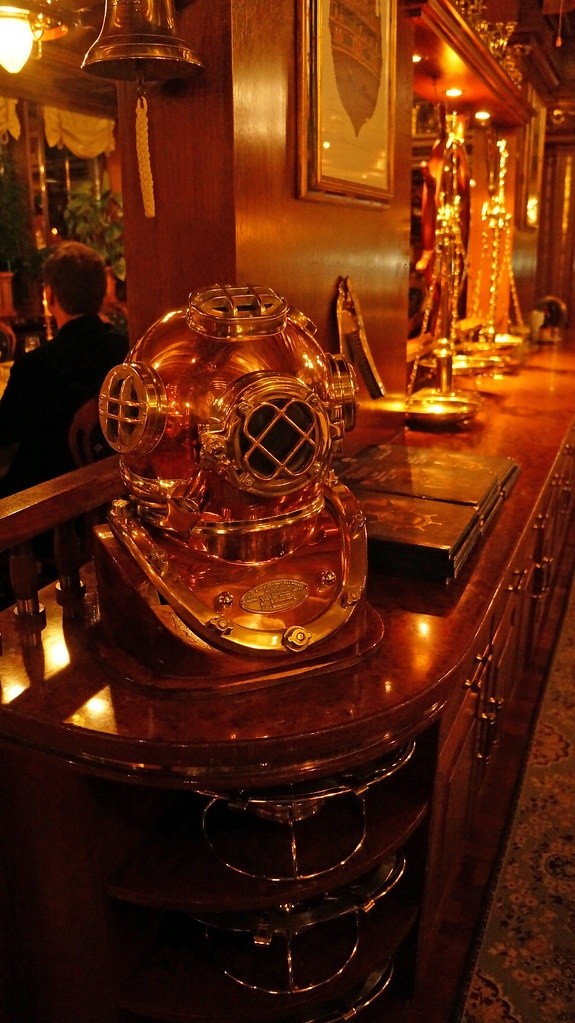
[0,0,78,74]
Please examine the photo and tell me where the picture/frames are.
[514,86,548,233]
[295,0,397,210]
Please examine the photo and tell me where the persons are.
[0,238,129,611]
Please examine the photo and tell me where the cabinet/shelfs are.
[0,416,575,1023]
